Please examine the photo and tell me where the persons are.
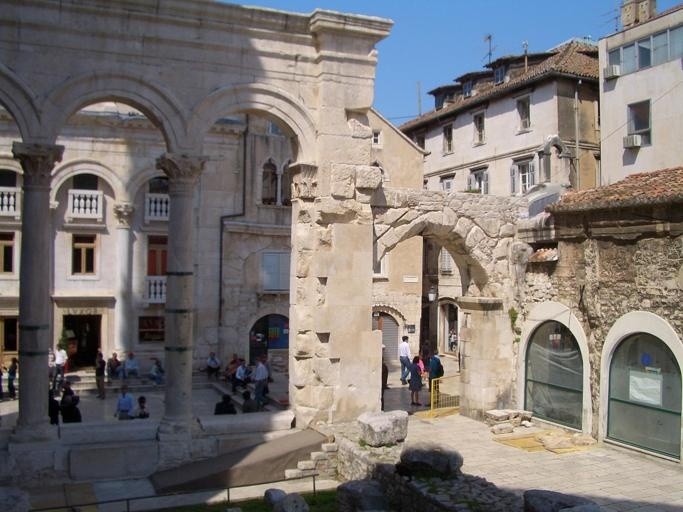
[4,357,19,401]
[47,341,273,424]
[382,336,444,410]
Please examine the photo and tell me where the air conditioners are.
[604,65,619,78]
[622,135,641,147]
